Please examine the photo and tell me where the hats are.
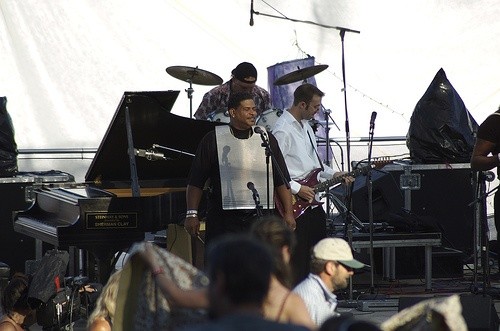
[314,237,371,272]
[232,62,258,84]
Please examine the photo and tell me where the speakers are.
[397,295,500,331]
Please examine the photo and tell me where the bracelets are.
[162,288,176,297]
[185,208,198,219]
[150,267,164,276]
[498,153,500,161]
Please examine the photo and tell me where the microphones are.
[250,0,254,26]
[370,112,377,134]
[254,126,266,134]
[246,181,259,204]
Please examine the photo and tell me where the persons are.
[270,83,356,286]
[471,107,500,270]
[0,301,31,331]
[194,62,270,126]
[183,92,297,276]
[85,214,381,331]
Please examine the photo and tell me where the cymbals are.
[166,66,224,86]
[273,64,328,86]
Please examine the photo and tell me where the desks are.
[353,232,442,292]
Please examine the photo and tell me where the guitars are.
[272,156,393,219]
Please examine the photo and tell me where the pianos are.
[11,90,189,308]
